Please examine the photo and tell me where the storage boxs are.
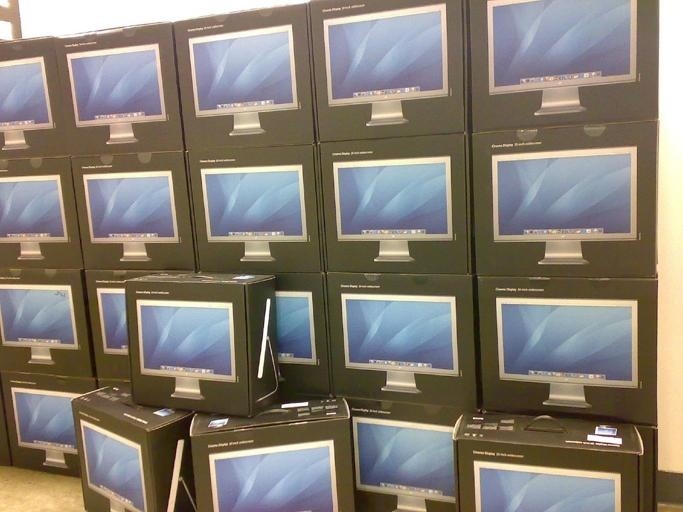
[123,274,278,420]
[465,0,660,124]
[54,24,187,155]
[0,269,95,380]
[184,144,322,271]
[336,395,467,512]
[69,151,196,273]
[0,39,69,163]
[72,386,194,511]
[314,132,472,274]
[273,271,326,406]
[470,119,660,278]
[474,274,658,430]
[5,380,96,479]
[189,399,356,510]
[174,4,318,151]
[455,411,659,511]
[85,268,193,393]
[322,270,478,422]
[0,157,83,270]
[309,0,469,144]
